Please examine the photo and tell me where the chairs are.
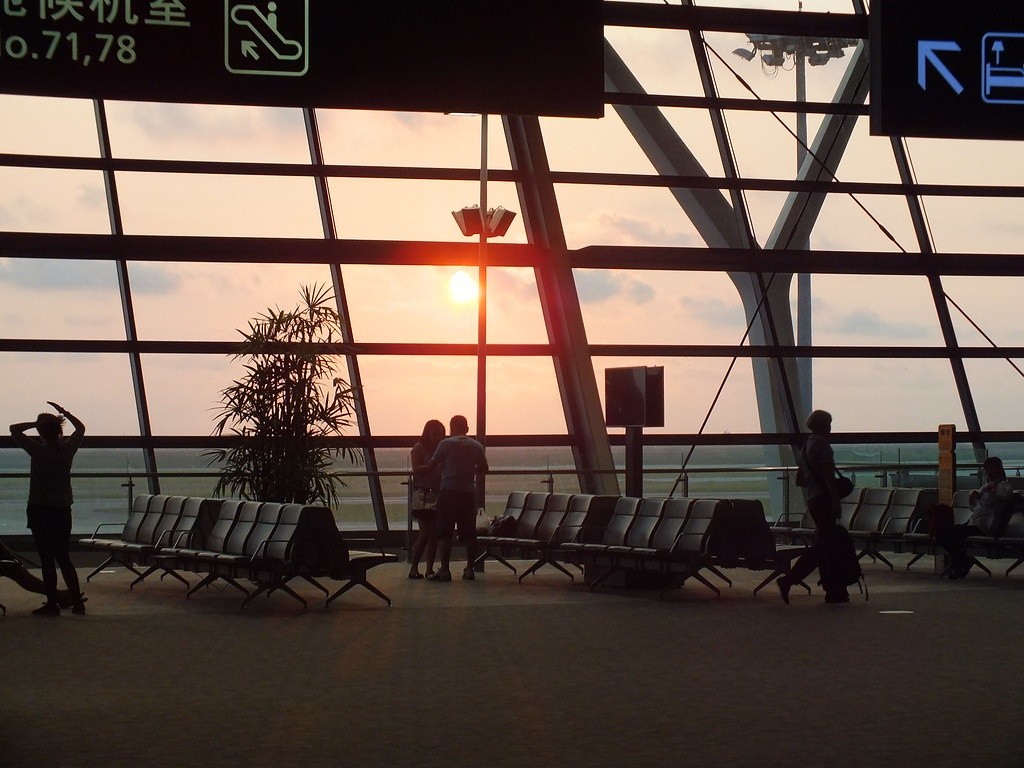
[463,472,1024,602]
[78,494,395,612]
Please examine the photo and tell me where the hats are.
[807,410,832,427]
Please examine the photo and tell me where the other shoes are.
[433,569,451,581]
[948,556,976,579]
[32,601,60,616]
[462,569,474,580]
[425,571,435,579]
[409,571,423,578]
[72,604,85,614]
[777,578,790,604]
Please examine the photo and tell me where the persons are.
[409,416,488,582]
[938,457,1013,581]
[776,410,844,604]
[8,401,87,616]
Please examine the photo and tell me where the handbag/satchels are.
[814,521,861,588]
[491,514,514,536]
[412,487,439,513]
[822,476,854,502]
[476,508,495,536]
